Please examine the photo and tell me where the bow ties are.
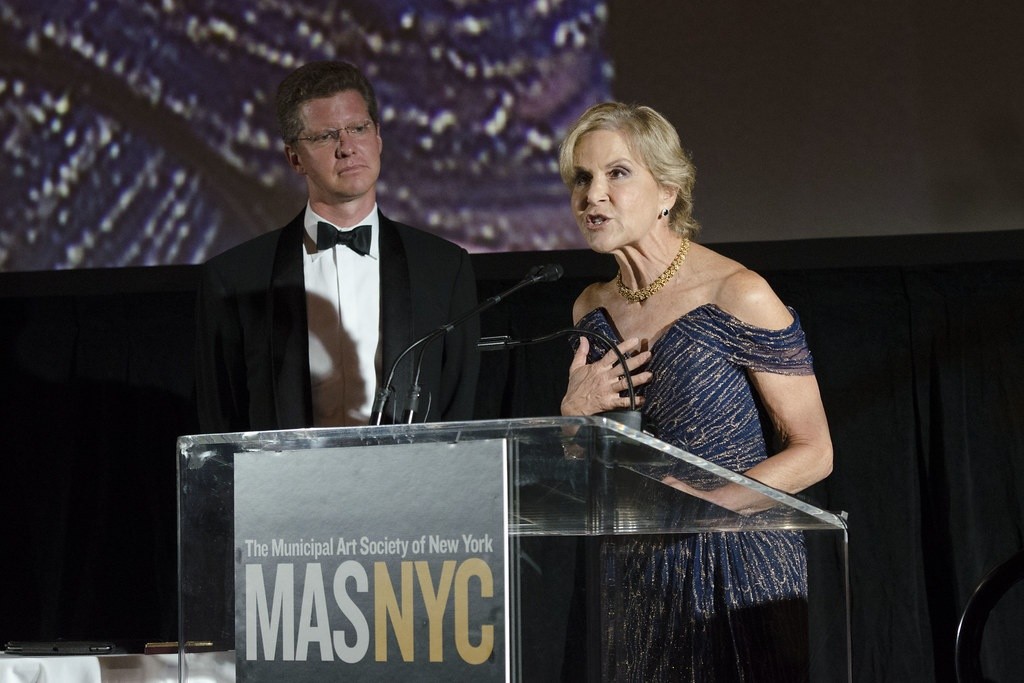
[314,220,372,257]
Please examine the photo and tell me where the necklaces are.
[616,233,691,303]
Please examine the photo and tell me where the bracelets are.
[563,445,586,460]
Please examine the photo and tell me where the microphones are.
[371,263,641,431]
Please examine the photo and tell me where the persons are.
[196,59,480,433]
[559,102,835,683]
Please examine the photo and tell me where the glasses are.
[291,113,376,146]
